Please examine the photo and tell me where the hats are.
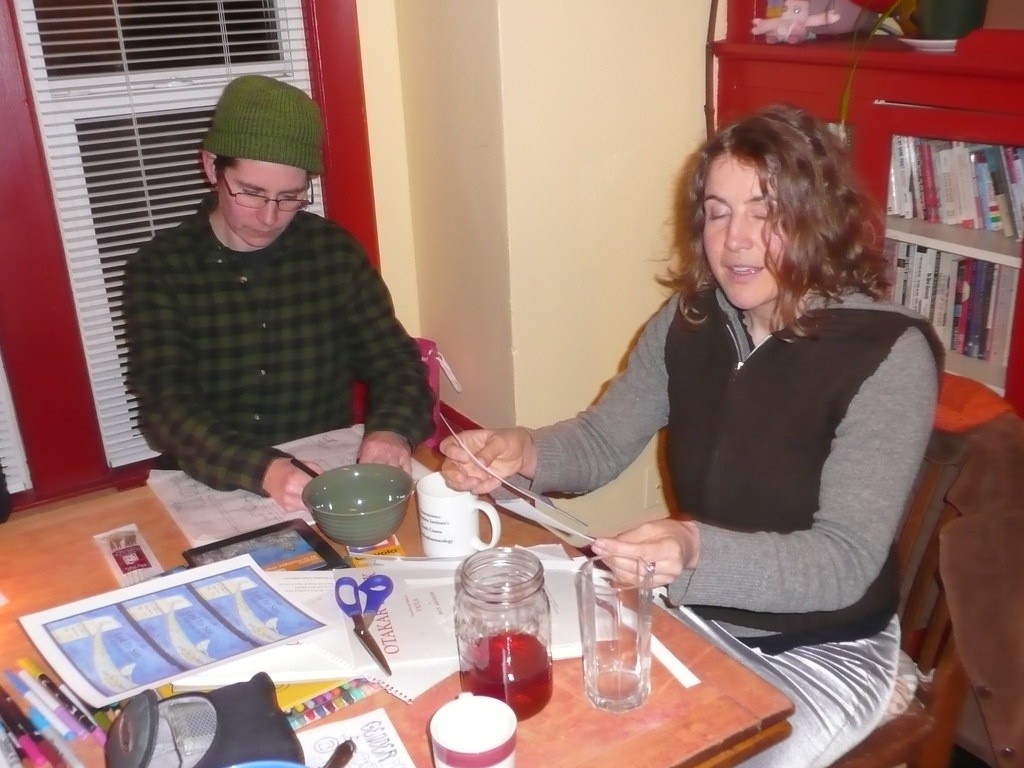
[203,74,325,177]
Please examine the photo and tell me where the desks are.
[1,442,795,768]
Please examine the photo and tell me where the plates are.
[896,32,958,52]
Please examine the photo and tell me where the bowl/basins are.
[302,464,414,546]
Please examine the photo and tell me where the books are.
[893,242,1020,368]
[826,123,1024,240]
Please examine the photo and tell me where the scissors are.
[335,575,395,676]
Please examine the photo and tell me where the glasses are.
[220,169,314,211]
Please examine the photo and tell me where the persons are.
[0,465,10,524]
[439,104,944,768]
[123,75,437,513]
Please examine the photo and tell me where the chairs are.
[827,429,976,768]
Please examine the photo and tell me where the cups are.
[580,554,653,714]
[429,691,518,768]
[417,470,503,558]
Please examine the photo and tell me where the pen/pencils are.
[77,698,113,733]
[28,707,85,768]
[19,670,90,739]
[290,458,319,479]
[3,670,75,741]
[59,684,109,750]
[18,657,96,734]
[0,685,67,768]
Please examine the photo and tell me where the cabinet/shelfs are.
[711,0,1023,419]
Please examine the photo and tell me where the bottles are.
[455,547,553,721]
